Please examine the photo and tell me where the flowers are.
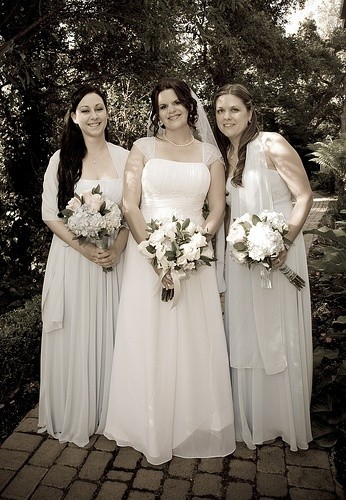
[225,208,306,291]
[59,184,130,274]
[136,215,218,302]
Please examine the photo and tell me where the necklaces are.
[88,154,102,163]
[163,132,195,147]
[230,154,235,160]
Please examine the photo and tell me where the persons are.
[103,77,236,465]
[37,83,130,448]
[207,84,313,451]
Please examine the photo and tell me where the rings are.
[278,258,280,262]
[108,258,111,262]
